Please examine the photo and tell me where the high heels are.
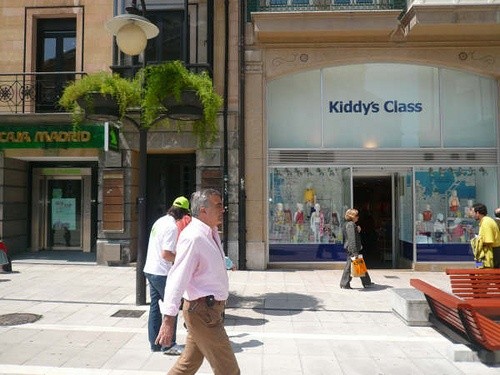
[364,283,374,288]
[340,283,352,289]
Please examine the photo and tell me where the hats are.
[173,196,190,211]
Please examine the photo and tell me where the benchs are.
[411,268,500,351]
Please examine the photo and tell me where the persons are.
[283,204,293,234]
[311,204,324,243]
[449,218,464,242]
[275,204,284,240]
[155,188,240,375]
[303,182,316,216]
[471,204,500,299]
[423,204,433,232]
[465,200,473,218]
[293,203,304,240]
[449,190,460,217]
[434,213,446,243]
[339,209,375,289]
[144,197,232,356]
[416,213,426,235]
[64,226,70,247]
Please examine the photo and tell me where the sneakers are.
[163,344,184,355]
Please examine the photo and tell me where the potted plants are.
[60,60,224,155]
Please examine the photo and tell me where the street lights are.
[105,0,159,305]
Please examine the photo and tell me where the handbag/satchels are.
[350,254,367,277]
